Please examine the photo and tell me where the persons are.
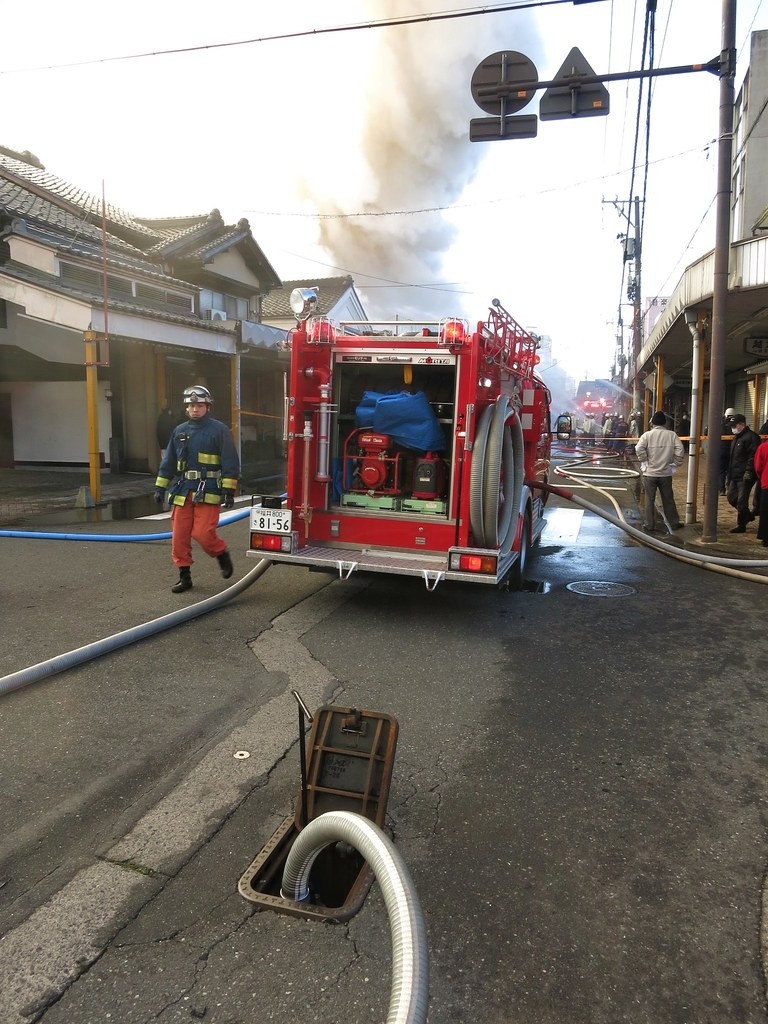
[634,411,685,530]
[555,410,644,454]
[677,415,690,448]
[704,404,768,548]
[153,387,240,596]
[155,408,178,460]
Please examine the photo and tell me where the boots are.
[217,552,233,579]
[172,566,192,592]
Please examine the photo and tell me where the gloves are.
[669,463,677,473]
[743,472,752,481]
[224,494,234,509]
[154,491,163,504]
[640,461,648,471]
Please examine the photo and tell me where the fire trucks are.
[242,285,554,586]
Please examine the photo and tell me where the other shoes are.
[730,510,768,547]
[642,523,684,532]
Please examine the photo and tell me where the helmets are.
[183,386,213,404]
[725,408,736,418]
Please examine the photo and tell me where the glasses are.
[183,387,207,398]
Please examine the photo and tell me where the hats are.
[586,412,642,418]
[725,414,746,426]
[651,411,666,425]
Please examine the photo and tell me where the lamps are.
[289,286,319,331]
